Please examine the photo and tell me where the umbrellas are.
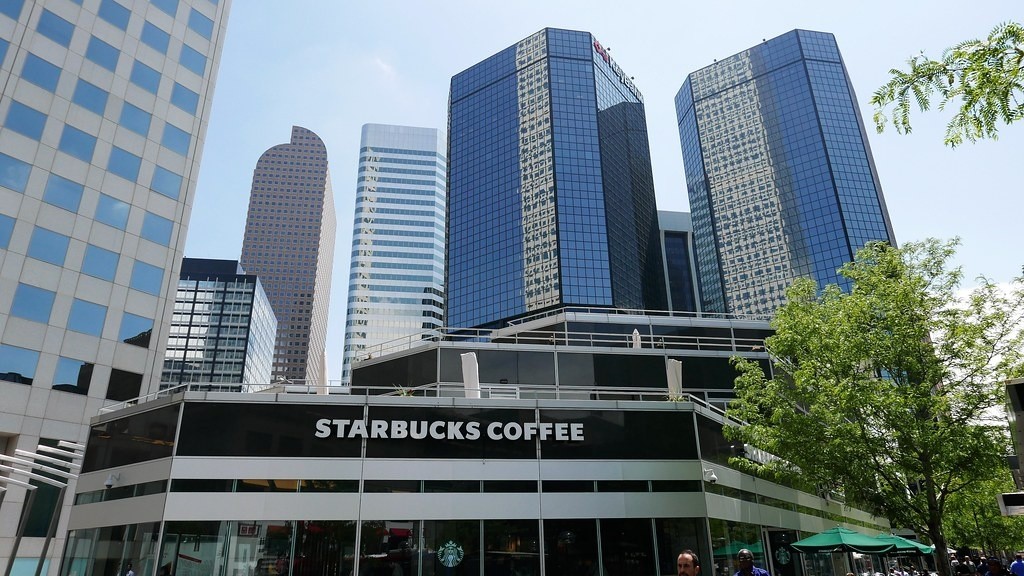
[790,524,956,576]
[713,538,763,555]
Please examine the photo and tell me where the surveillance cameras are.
[709,475,717,483]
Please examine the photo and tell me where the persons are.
[949,554,1024,576]
[677,549,700,576]
[126,564,135,576]
[733,549,770,576]
